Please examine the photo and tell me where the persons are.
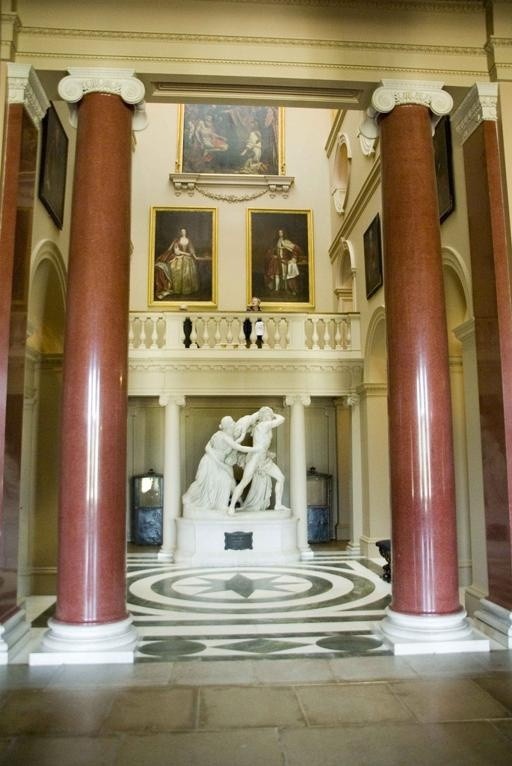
[171,226,200,294]
[181,416,261,508]
[227,406,291,513]
[240,130,262,169]
[246,297,264,311]
[233,411,259,444]
[195,115,229,156]
[273,227,296,290]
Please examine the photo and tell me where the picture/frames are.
[147,204,220,308]
[245,207,316,312]
[175,102,285,176]
[363,211,383,301]
[38,100,70,231]
[434,114,456,224]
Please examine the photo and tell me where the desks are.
[376,539,392,583]
